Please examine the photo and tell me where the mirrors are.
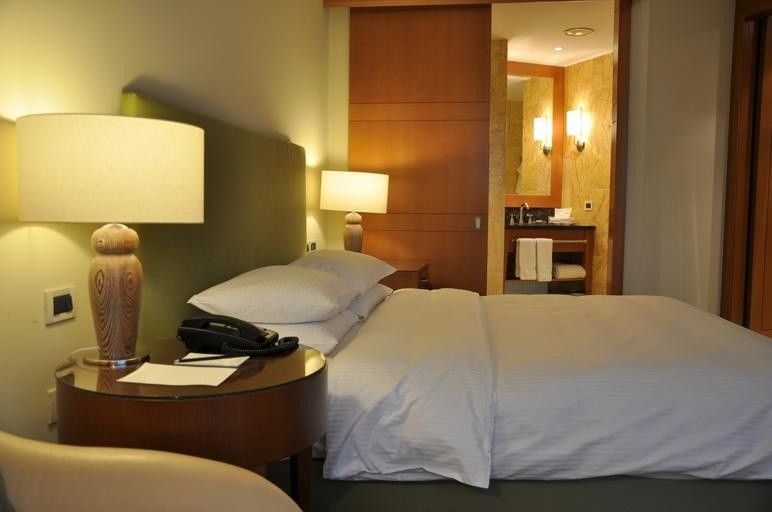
[504,60,565,209]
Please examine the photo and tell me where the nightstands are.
[379,260,430,292]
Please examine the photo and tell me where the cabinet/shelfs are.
[504,229,594,295]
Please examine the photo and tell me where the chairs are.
[1,431,302,511]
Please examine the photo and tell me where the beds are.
[119,91,772,511]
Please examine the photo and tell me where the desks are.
[52,337,326,510]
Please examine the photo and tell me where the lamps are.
[533,117,551,157]
[14,113,205,368]
[320,170,389,253]
[566,110,585,152]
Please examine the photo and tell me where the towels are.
[515,238,537,281]
[552,263,586,279]
[515,163,522,194]
[536,238,552,282]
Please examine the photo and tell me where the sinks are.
[514,224,562,229]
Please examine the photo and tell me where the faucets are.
[519,202,530,224]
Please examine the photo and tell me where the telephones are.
[176,315,279,353]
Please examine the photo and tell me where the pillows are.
[250,309,360,356]
[288,248,399,298]
[348,283,395,322]
[185,265,362,323]
